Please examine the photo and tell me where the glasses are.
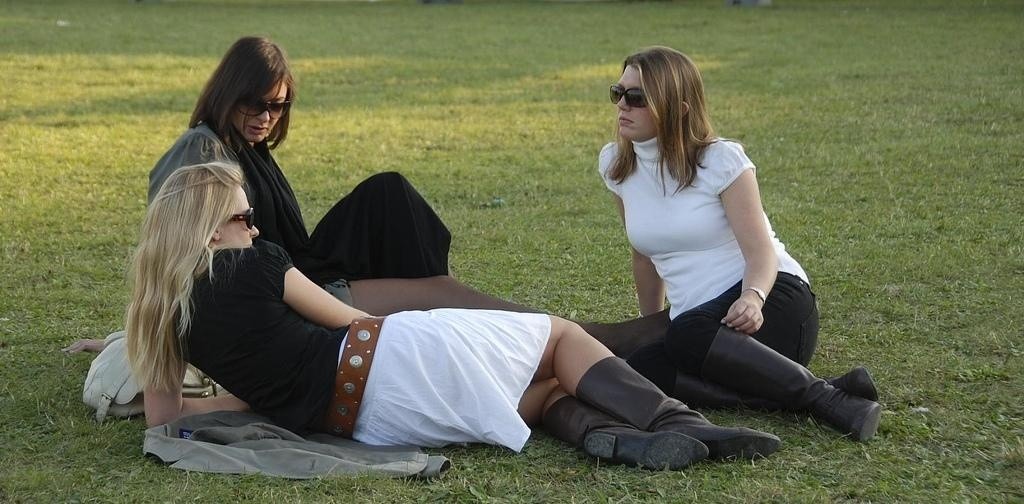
[237,97,290,118]
[610,84,648,107]
[230,208,254,229]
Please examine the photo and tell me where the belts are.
[323,317,383,437]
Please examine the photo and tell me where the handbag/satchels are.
[83,337,229,423]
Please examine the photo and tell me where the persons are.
[596,44,884,446]
[123,159,783,473]
[60,30,671,394]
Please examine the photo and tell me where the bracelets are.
[739,280,769,308]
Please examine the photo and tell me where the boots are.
[675,325,881,440]
[544,356,781,470]
